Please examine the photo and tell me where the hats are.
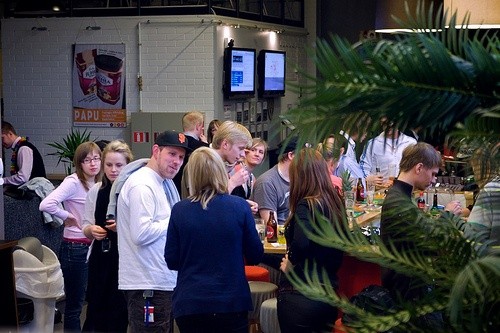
[155,129,189,150]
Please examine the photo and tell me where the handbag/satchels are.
[340,285,392,327]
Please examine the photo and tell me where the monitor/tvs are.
[225,47,256,95]
[257,50,286,93]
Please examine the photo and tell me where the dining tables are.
[262,202,383,254]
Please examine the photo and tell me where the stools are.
[248,281,278,333]
[259,297,282,333]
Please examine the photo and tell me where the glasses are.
[80,157,101,164]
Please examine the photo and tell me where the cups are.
[254,217,265,243]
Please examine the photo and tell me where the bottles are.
[356,177,364,201]
[431,193,438,214]
[142,298,157,333]
[417,192,425,211]
[375,166,382,191]
[267,211,278,243]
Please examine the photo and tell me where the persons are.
[117,129,189,332]
[403,118,423,141]
[316,142,341,194]
[277,148,348,333]
[463,141,499,254]
[81,138,128,333]
[322,133,364,193]
[171,112,208,202]
[225,136,268,199]
[39,139,104,333]
[1,120,46,199]
[253,136,312,225]
[338,109,382,187]
[379,141,456,331]
[165,145,264,332]
[206,120,258,216]
[206,119,222,146]
[359,110,417,185]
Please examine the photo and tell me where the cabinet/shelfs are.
[0,239,20,332]
[423,188,475,208]
